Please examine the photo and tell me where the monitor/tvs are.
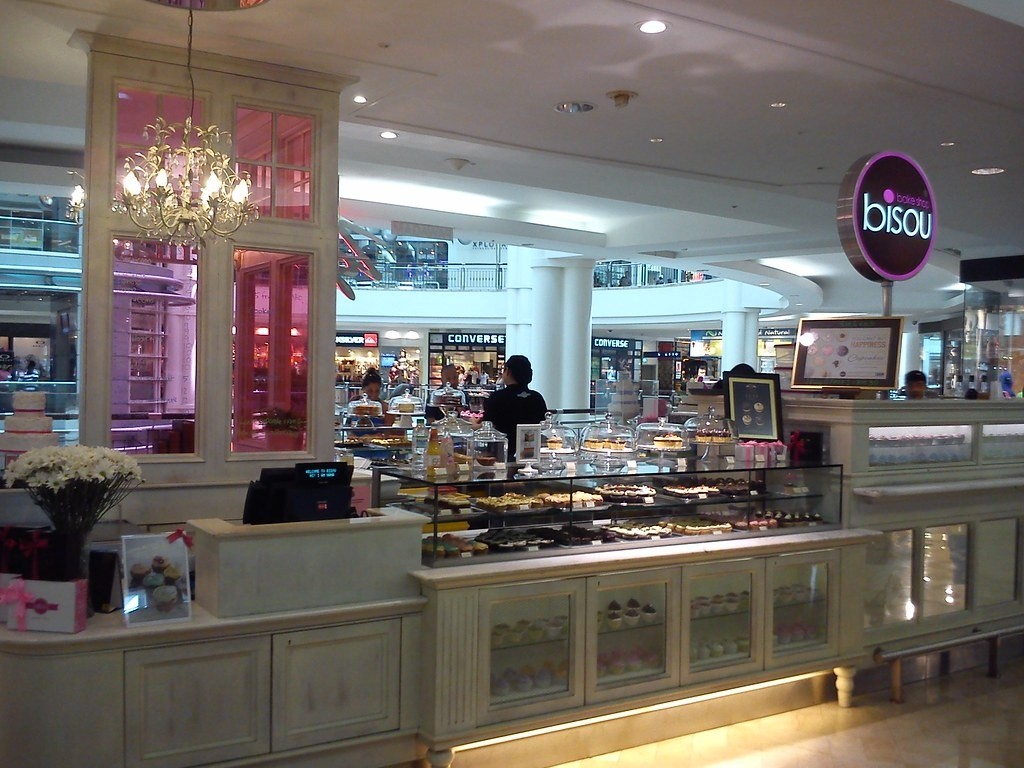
[243,462,354,526]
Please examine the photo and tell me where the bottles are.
[966,375,978,399]
[429,423,442,441]
[467,421,508,471]
[442,439,454,476]
[954,376,964,398]
[979,376,989,400]
[424,429,446,476]
[412,419,428,476]
[432,411,474,474]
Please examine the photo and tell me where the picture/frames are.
[120,531,197,631]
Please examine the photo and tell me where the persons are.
[459,369,504,384]
[965,389,978,399]
[484,355,547,458]
[619,271,630,286]
[0,361,40,392]
[350,368,395,437]
[905,370,926,399]
[390,384,414,399]
[656,277,664,285]
[680,376,708,391]
[428,364,470,419]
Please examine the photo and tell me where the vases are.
[50,528,95,619]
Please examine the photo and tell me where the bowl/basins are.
[598,663,660,677]
[597,609,657,629]
[493,621,566,645]
[773,633,817,646]
[774,588,818,603]
[491,679,566,695]
[690,596,749,617]
[690,643,748,661]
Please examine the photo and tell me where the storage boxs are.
[12,572,89,636]
[0,572,21,624]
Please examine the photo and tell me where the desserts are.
[334,433,414,448]
[404,428,823,561]
[131,554,181,610]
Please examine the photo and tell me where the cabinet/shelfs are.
[0,295,1024,768]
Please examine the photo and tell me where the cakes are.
[354,405,379,416]
[608,381,639,413]
[0,391,59,452]
[399,403,414,413]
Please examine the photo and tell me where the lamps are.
[65,168,87,227]
[121,0,270,248]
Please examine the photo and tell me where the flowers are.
[2,443,147,540]
